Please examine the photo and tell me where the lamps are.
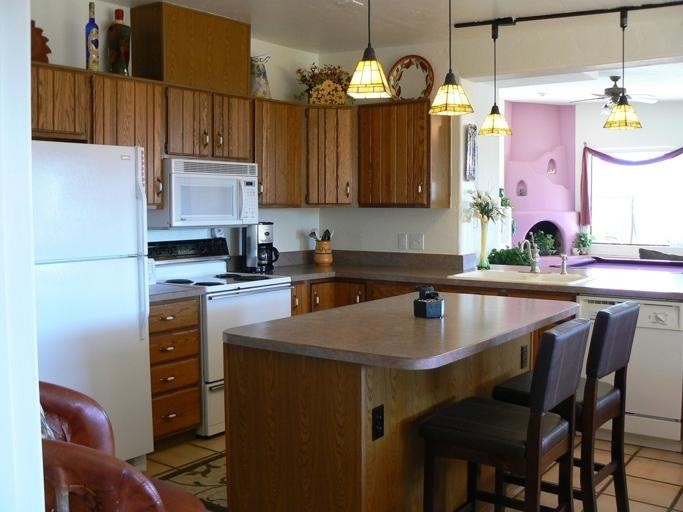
[427,0,473,116]
[600,97,621,113]
[346,0,392,99]
[453,0,683,135]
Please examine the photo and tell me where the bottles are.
[105,9,133,77]
[84,2,100,73]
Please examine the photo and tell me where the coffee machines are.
[247,221,279,273]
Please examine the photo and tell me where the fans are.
[568,76,659,104]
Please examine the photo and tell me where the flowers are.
[294,62,353,104]
[464,187,507,224]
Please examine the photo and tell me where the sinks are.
[528,274,594,285]
[447,269,529,284]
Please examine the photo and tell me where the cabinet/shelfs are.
[149,299,202,447]
[253,96,304,209]
[92,70,164,208]
[32,65,90,138]
[306,105,356,206]
[107,34,252,95]
[354,99,448,208]
[165,82,253,160]
[291,282,575,372]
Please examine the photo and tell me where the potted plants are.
[574,231,595,255]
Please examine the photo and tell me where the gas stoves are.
[153,271,292,292]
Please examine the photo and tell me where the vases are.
[477,220,491,270]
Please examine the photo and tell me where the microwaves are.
[159,156,263,229]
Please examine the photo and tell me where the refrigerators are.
[28,141,156,464]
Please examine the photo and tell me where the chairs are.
[492,300,640,512]
[42,438,165,512]
[39,381,207,512]
[419,318,589,512]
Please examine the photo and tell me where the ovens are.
[197,286,293,440]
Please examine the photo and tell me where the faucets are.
[520,239,541,273]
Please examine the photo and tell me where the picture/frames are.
[465,124,477,181]
